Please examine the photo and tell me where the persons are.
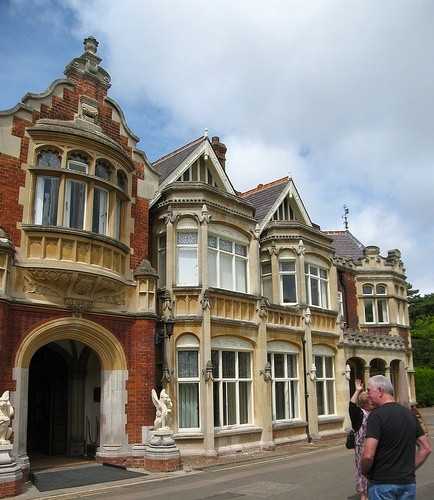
[359,374,433,500]
[349,378,375,500]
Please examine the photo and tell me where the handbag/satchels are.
[346,429,355,449]
